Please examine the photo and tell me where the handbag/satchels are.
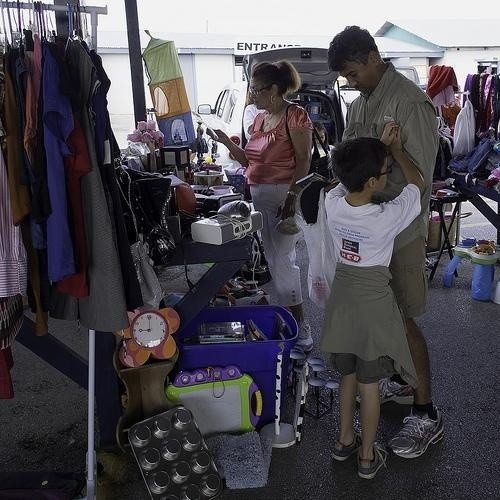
[112,164,177,266]
[114,161,163,312]
[428,211,472,250]
[309,154,335,181]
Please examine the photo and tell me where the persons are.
[328,24,446,460]
[318,122,426,480]
[311,121,336,171]
[206,60,315,360]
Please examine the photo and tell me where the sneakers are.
[333,433,363,461]
[298,321,313,351]
[358,442,389,480]
[355,376,414,406]
[390,407,446,460]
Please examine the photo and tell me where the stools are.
[430,190,465,285]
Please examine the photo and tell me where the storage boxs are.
[180,304,298,419]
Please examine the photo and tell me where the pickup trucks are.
[337,64,429,108]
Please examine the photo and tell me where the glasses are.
[248,84,271,96]
[381,166,392,177]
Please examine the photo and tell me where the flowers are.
[130,120,166,147]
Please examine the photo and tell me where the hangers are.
[2,1,92,51]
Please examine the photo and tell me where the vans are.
[193,42,347,166]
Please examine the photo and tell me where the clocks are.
[110,299,180,367]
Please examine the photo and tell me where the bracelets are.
[286,191,297,197]
